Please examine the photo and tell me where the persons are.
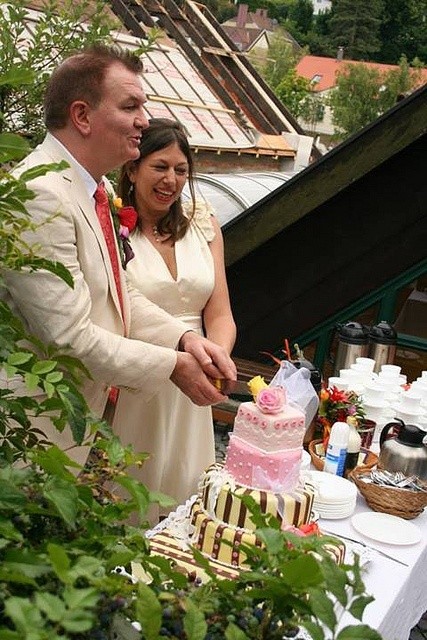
[0,40,239,480]
[112,119,237,532]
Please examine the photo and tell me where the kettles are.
[378,418,427,480]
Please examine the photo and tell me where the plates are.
[351,512,421,546]
[298,470,357,519]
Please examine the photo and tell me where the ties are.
[93,182,125,405]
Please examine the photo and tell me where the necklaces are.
[147,223,162,244]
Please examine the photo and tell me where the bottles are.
[343,416,360,470]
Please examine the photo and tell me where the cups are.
[329,357,427,417]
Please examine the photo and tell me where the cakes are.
[131,374,345,594]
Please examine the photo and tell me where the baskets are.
[350,468,426,520]
[307,438,378,479]
